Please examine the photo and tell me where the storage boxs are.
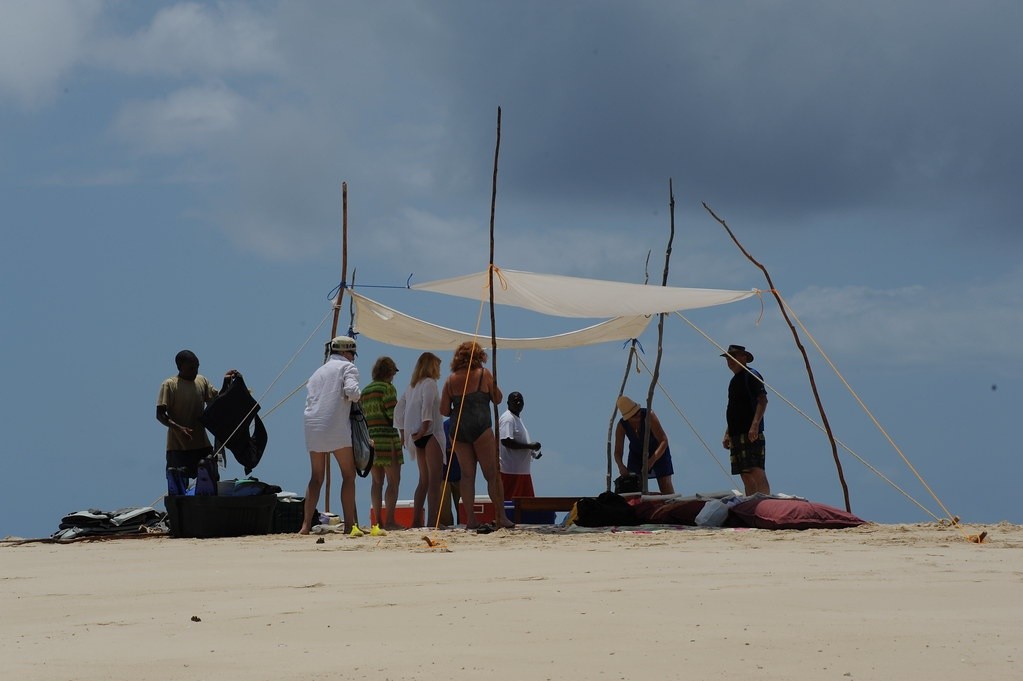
[456,494,495,526]
[370,500,426,529]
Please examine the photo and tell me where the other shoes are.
[477,522,491,534]
[488,519,499,531]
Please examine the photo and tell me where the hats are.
[719,344,754,363]
[616,395,641,421]
[330,335,357,353]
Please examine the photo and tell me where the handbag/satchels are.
[596,490,640,527]
[694,497,730,528]
[348,401,375,478]
[572,497,603,527]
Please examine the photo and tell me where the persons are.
[439,342,515,529]
[442,417,461,525]
[358,356,408,530]
[297,337,370,534]
[156,349,235,494]
[497,391,543,500]
[392,352,453,529]
[720,345,770,497]
[614,396,675,494]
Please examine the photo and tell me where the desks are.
[511,496,579,524]
